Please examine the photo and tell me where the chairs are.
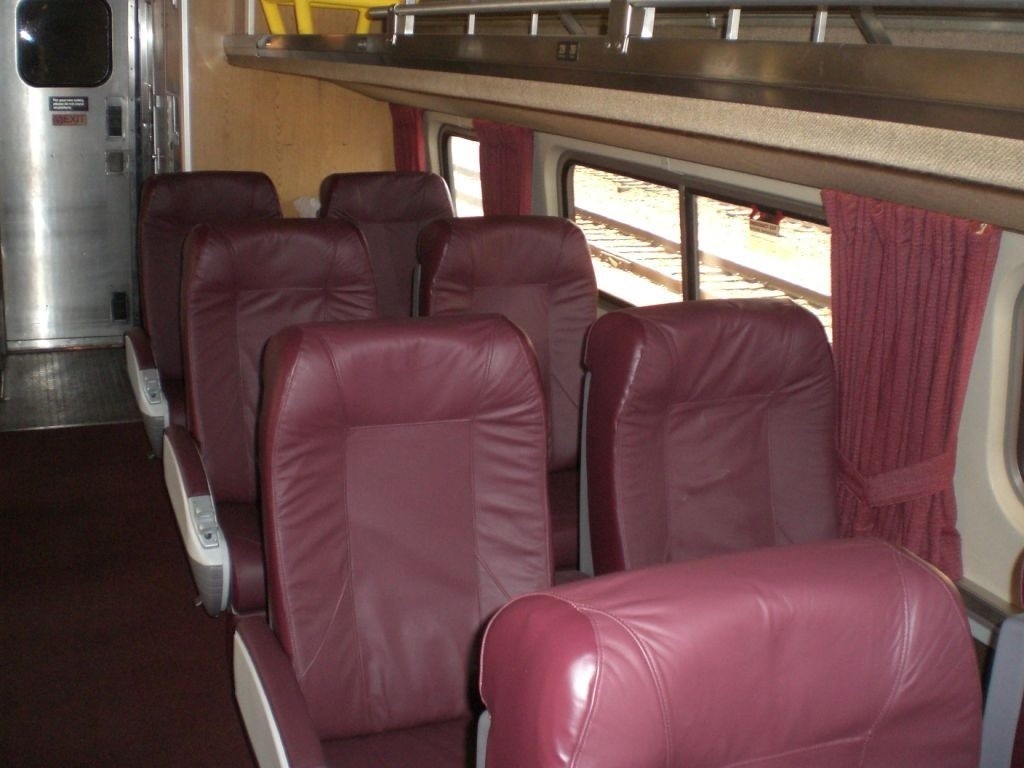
[123,170,1023,768]
